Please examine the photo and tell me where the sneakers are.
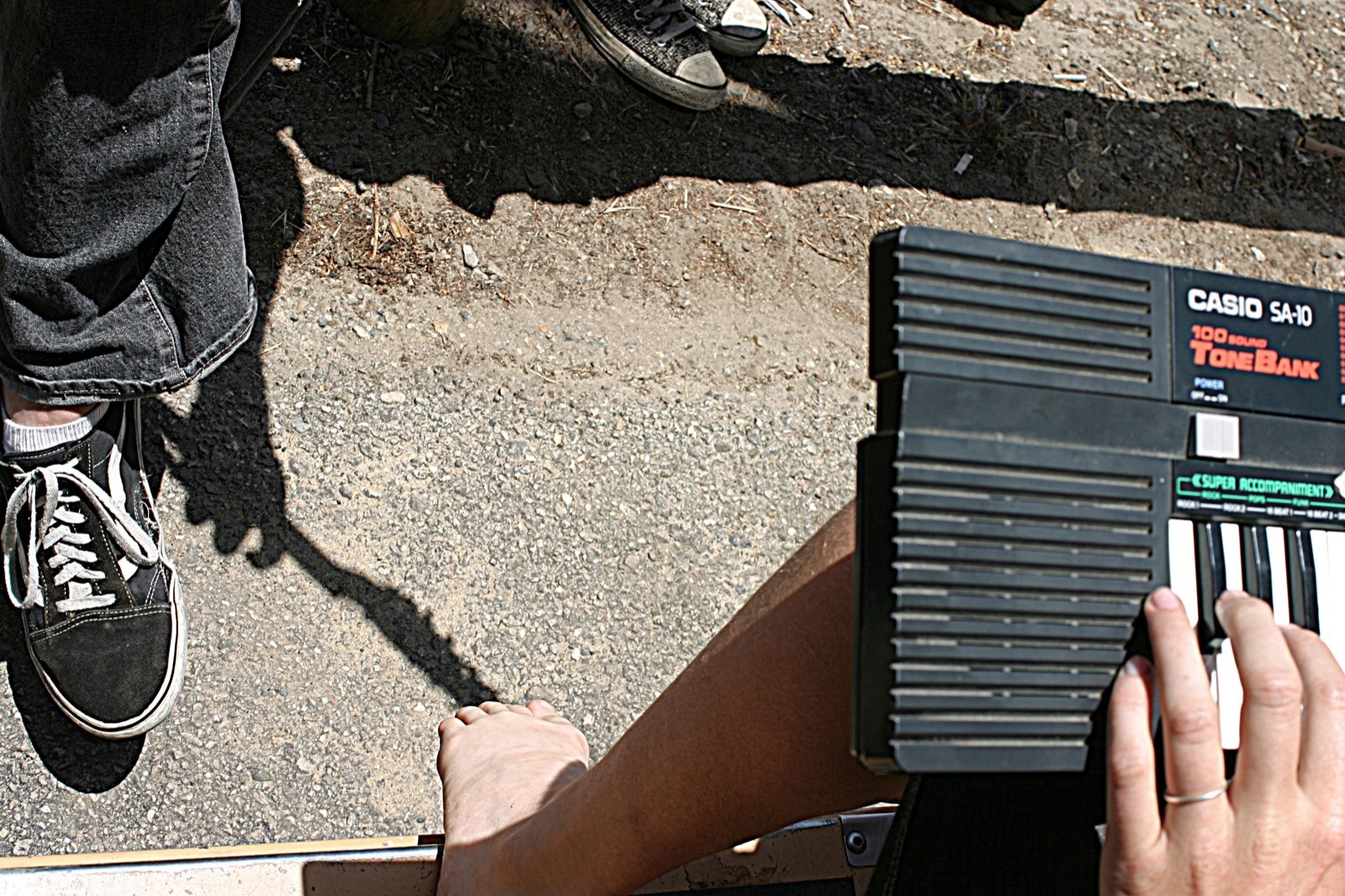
[572,0,729,112]
[689,1,769,61]
[0,398,186,740]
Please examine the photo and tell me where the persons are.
[571,0,768,112]
[0,0,258,740]
[439,498,1345,896]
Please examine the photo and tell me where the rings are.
[1164,783,1227,802]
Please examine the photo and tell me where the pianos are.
[848,225,1345,779]
